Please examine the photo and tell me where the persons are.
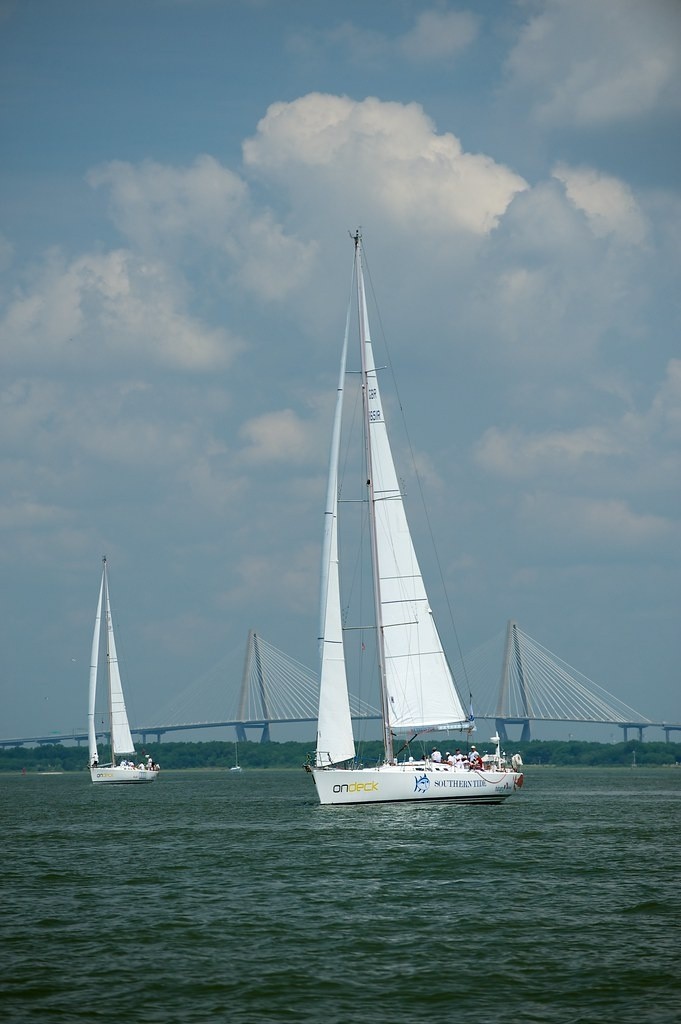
[446,752,457,765]
[120,756,160,771]
[91,753,98,768]
[431,747,441,763]
[510,750,523,773]
[453,748,463,761]
[468,746,479,762]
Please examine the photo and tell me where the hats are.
[456,748,460,751]
[446,751,451,756]
[432,747,437,750]
[471,745,476,749]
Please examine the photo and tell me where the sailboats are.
[301,229,525,806]
[88,557,160,782]
[230,743,242,771]
